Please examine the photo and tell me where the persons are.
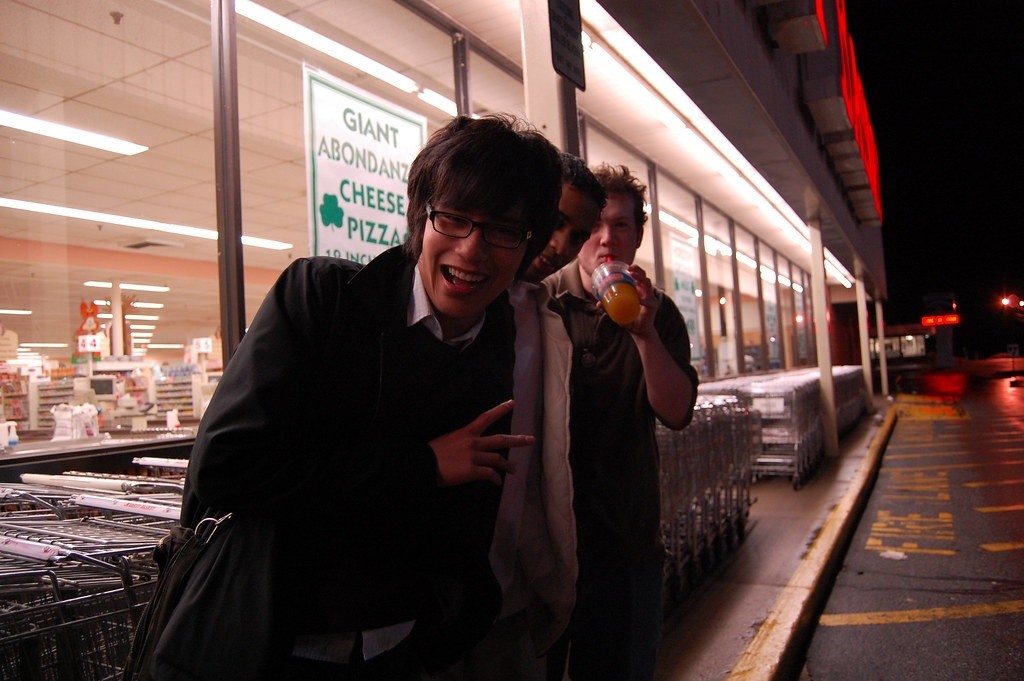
[180,116,698,681]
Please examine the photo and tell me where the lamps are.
[581,26,852,288]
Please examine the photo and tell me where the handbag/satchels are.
[120,512,269,681]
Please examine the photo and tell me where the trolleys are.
[0,456,192,681]
[654,361,867,616]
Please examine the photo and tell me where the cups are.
[591,261,640,324]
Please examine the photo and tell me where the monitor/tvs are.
[89,375,117,401]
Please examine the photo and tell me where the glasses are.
[425,201,533,250]
